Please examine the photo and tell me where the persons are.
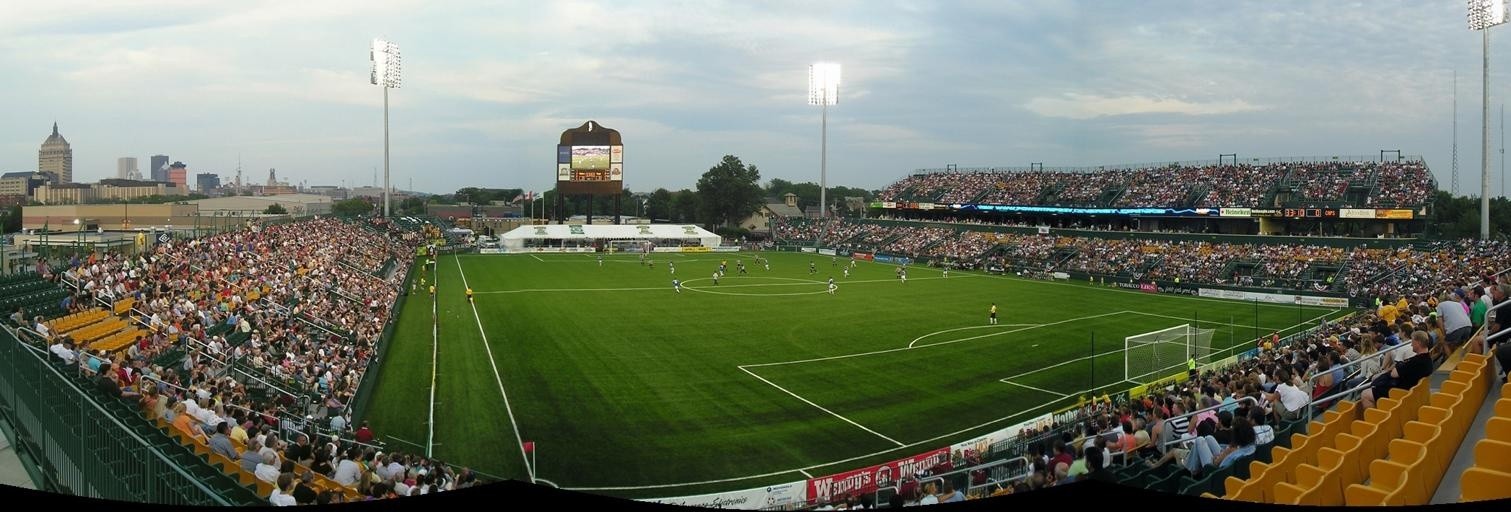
[9,212,482,508]
[669,262,674,274]
[733,159,1509,512]
[371,201,381,219]
[642,238,657,252]
[640,253,653,270]
[672,277,681,296]
[713,260,727,286]
[597,255,603,268]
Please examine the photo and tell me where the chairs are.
[1,271,276,506]
[1112,342,1511,507]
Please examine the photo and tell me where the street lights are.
[73,218,86,246]
[808,62,841,220]
[1469,0,1511,242]
[370,38,400,216]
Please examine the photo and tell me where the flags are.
[523,442,533,453]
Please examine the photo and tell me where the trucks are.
[477,235,496,248]
[449,228,476,243]
